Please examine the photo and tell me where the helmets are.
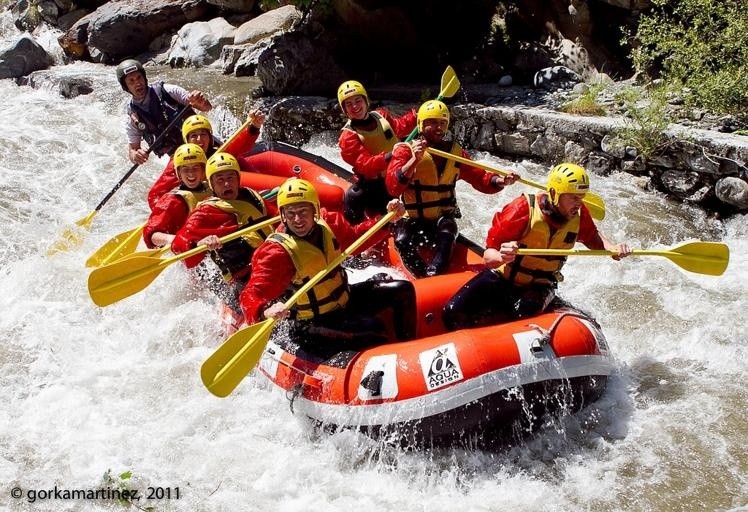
[337,80,370,115]
[547,163,591,206]
[173,143,210,180]
[277,180,321,227]
[116,59,146,95]
[205,152,240,190]
[181,115,213,143]
[416,99,450,135]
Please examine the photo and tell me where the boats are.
[179,142,615,448]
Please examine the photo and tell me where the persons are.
[117,60,631,361]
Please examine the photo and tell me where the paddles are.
[85,115,252,267]
[87,185,286,309]
[201,208,396,397]
[47,99,194,261]
[414,140,606,221]
[388,64,460,159]
[513,242,729,275]
